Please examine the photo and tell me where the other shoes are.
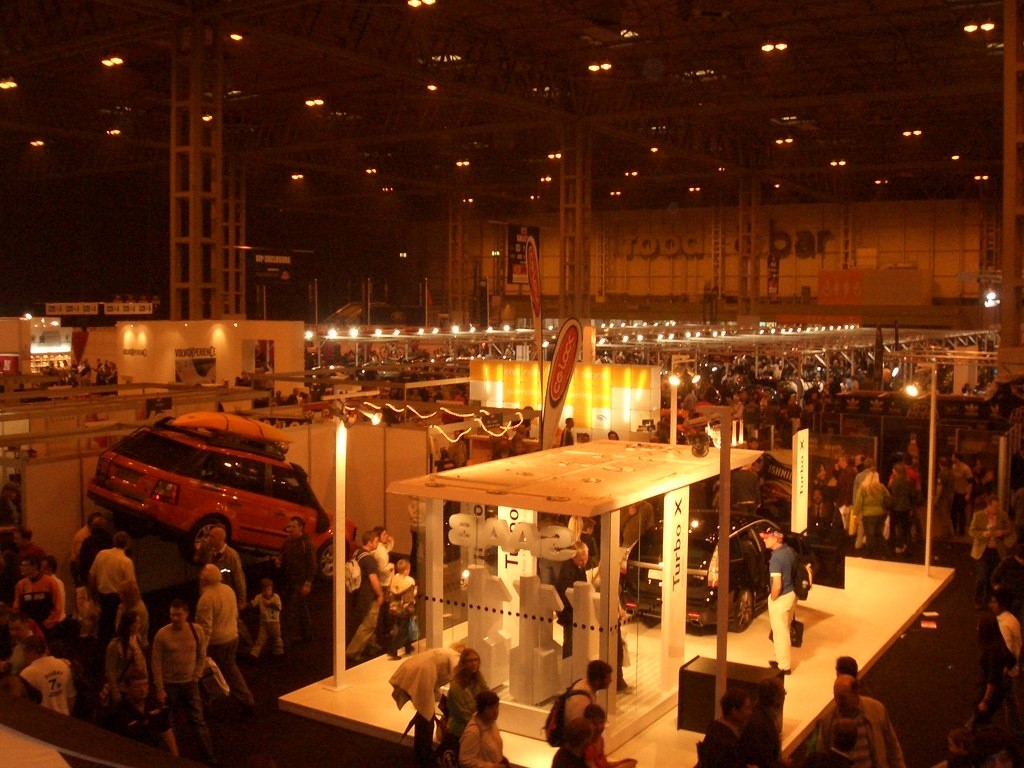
[617,686,636,694]
[768,662,791,675]
[975,602,982,610]
[406,646,414,654]
[386,647,401,660]
[896,544,906,553]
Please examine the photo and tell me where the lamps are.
[357,409,382,425]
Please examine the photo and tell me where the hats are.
[759,526,785,538]
[991,583,1014,605]
[10,474,22,481]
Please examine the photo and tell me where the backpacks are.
[345,548,370,594]
[788,546,812,600]
[542,678,592,747]
[431,724,482,768]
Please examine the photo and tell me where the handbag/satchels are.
[839,505,857,535]
[769,612,803,647]
[882,515,890,540]
[200,656,231,701]
[1004,530,1017,548]
[881,487,895,511]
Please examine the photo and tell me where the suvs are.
[87,412,358,579]
[621,508,847,636]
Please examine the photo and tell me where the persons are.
[389,646,510,768]
[331,336,923,570]
[254,355,273,408]
[555,540,589,658]
[694,657,905,768]
[758,526,796,676]
[933,447,1024,768]
[271,351,338,429]
[36,359,118,400]
[0,481,417,762]
[0,365,7,404]
[552,659,637,768]
[586,560,636,694]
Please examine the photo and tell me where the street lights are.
[311,276,497,328]
